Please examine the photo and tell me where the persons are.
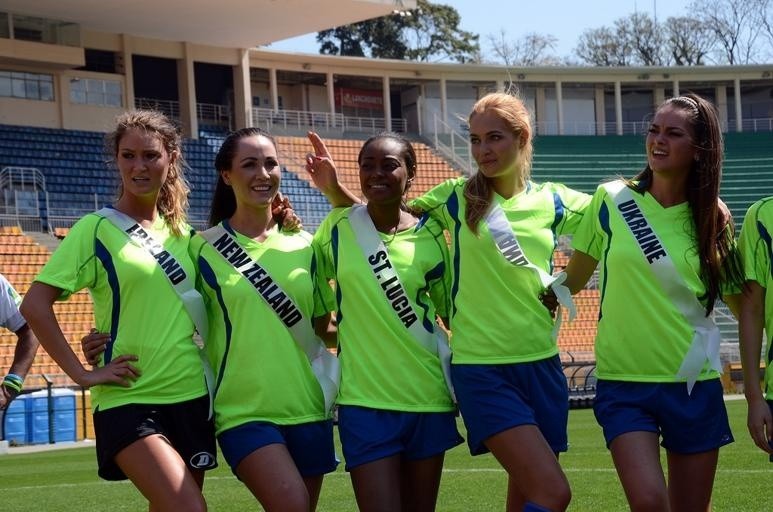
[304,91,609,512]
[736,195,771,476]
[301,127,563,512]
[19,107,304,512]
[545,90,744,512]
[75,126,343,512]
[0,272,41,414]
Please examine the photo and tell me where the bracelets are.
[1,373,24,398]
